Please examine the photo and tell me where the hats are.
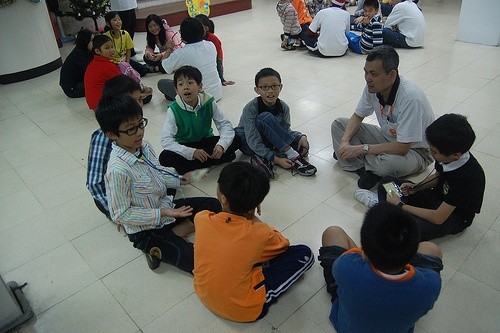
[331,0,346,6]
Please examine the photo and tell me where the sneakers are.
[288,154,317,176]
[353,189,379,208]
[251,155,275,179]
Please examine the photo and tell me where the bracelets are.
[397,201,405,209]
[362,144,369,157]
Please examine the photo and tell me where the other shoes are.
[143,252,160,269]
[282,43,297,50]
[333,152,337,159]
[228,153,236,161]
[358,173,381,190]
[143,64,156,72]
[130,55,143,62]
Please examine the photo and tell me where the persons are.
[331,44,436,189]
[318,201,443,333]
[233,67,317,176]
[94,90,223,276]
[193,160,315,323]
[110,0,138,57]
[276,0,426,58]
[85,73,176,232]
[354,113,485,242]
[59,11,235,104]
[159,65,241,175]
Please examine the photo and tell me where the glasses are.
[258,85,280,90]
[171,32,183,46]
[118,118,147,136]
